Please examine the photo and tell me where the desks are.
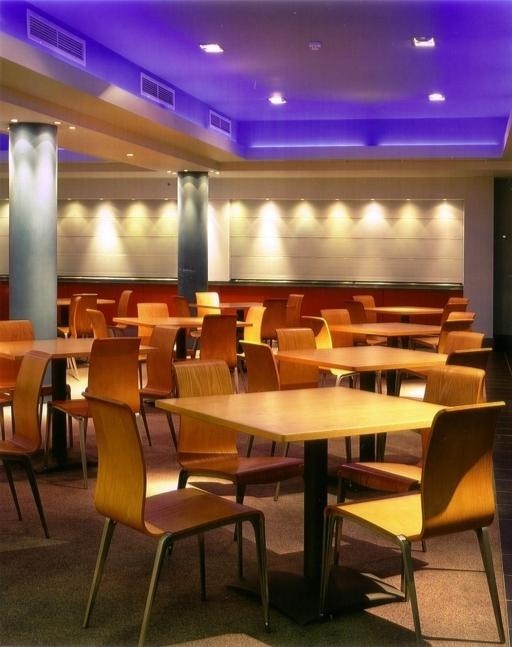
[157,387,450,627]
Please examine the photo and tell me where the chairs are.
[320,402,505,647]
[171,361,304,597]
[1,289,494,389]
[81,392,272,646]
[2,350,53,541]
[331,365,486,599]
[54,339,142,490]
